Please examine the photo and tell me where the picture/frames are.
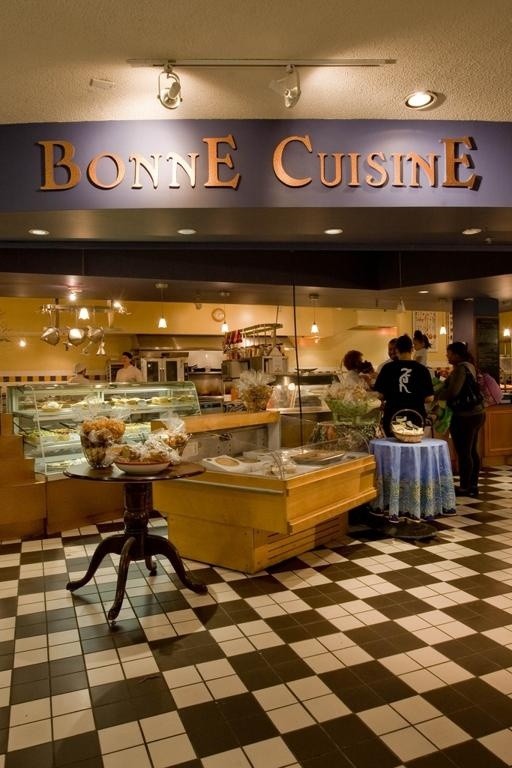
[411,309,439,354]
[446,311,454,346]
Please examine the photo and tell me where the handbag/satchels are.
[476,369,504,407]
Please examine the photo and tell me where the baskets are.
[391,408,424,442]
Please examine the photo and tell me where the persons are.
[67,362,92,385]
[114,351,143,384]
[374,337,397,375]
[341,349,370,391]
[372,334,435,439]
[440,341,487,497]
[356,360,379,388]
[412,330,430,368]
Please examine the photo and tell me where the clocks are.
[211,307,226,323]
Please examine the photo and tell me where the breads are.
[21,396,169,443]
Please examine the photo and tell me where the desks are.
[61,460,211,629]
[314,419,378,455]
[365,436,457,542]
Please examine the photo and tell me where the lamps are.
[396,251,406,313]
[125,57,394,112]
[310,292,319,334]
[156,279,168,329]
[78,245,91,322]
[436,297,447,336]
[220,289,231,333]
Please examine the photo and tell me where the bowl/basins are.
[116,461,169,474]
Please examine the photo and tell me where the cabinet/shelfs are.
[149,408,380,576]
[266,372,341,447]
[4,379,201,535]
[141,357,184,383]
